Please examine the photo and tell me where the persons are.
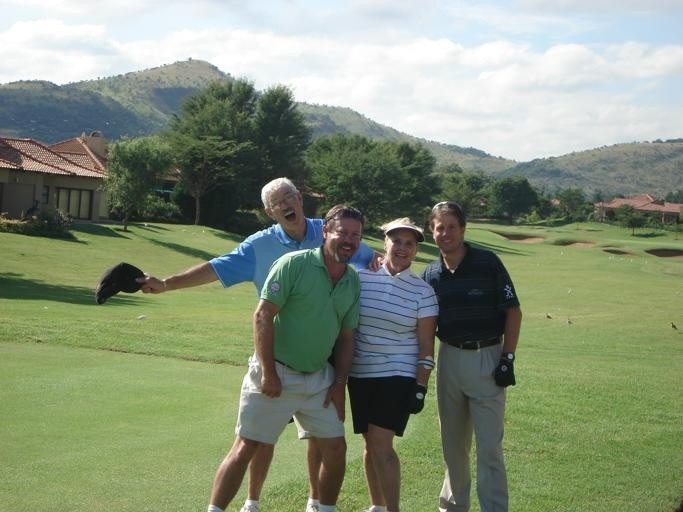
[420,201,522,512]
[132,178,383,512]
[351,217,439,512]
[206,205,367,512]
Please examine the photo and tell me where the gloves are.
[408,383,427,414]
[493,356,516,388]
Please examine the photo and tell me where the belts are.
[445,338,501,350]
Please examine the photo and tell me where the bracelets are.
[333,374,346,385]
[160,278,168,291]
[416,358,435,369]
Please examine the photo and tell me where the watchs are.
[501,352,515,361]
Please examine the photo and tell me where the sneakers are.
[239,505,260,512]
[305,504,320,512]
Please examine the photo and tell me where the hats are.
[96,262,145,305]
[384,221,424,243]
